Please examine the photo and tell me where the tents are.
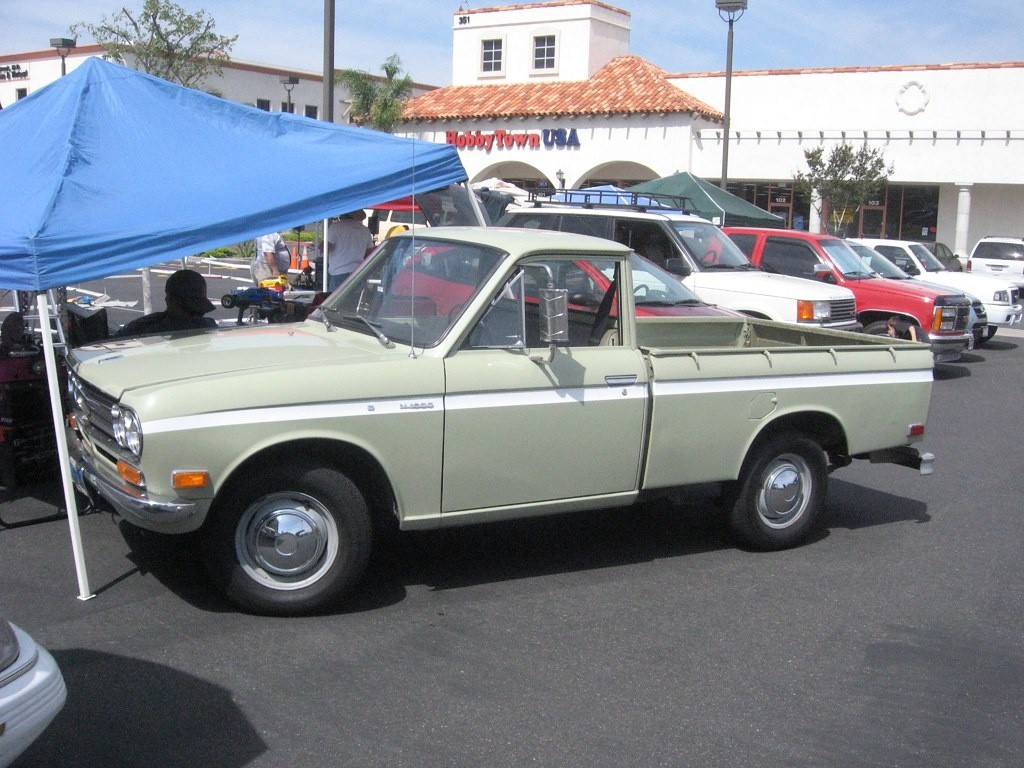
[541,171,788,233]
[0,54,514,601]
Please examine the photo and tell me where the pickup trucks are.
[61,223,941,617]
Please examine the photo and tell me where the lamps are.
[622,112,631,119]
[660,113,666,119]
[569,115,578,121]
[1007,130,1011,139]
[458,118,466,123]
[472,117,482,123]
[886,131,891,138]
[535,116,544,121]
[586,114,595,120]
[702,115,711,122]
[777,132,782,138]
[981,130,985,138]
[714,117,719,123]
[414,119,423,124]
[520,116,528,121]
[863,131,868,137]
[697,132,700,137]
[909,131,914,138]
[716,132,720,138]
[689,111,699,120]
[503,116,512,122]
[641,112,648,118]
[555,169,564,189]
[736,132,739,138]
[798,131,803,137]
[552,114,561,120]
[427,119,437,125]
[841,131,845,138]
[819,130,824,138]
[957,131,961,138]
[756,132,761,137]
[488,118,495,123]
[932,131,937,138]
[604,113,613,120]
[443,118,452,124]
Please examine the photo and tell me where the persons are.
[250,232,292,285]
[114,269,218,338]
[318,210,372,316]
[380,225,412,287]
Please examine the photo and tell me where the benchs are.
[479,302,620,348]
[308,292,437,317]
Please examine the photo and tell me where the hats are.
[165,269,216,315]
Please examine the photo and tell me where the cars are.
[391,237,763,324]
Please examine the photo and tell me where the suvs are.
[704,224,1024,362]
[482,185,864,338]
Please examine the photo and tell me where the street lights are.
[714,1,749,190]
[49,37,76,77]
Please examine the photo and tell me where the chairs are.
[639,240,669,270]
